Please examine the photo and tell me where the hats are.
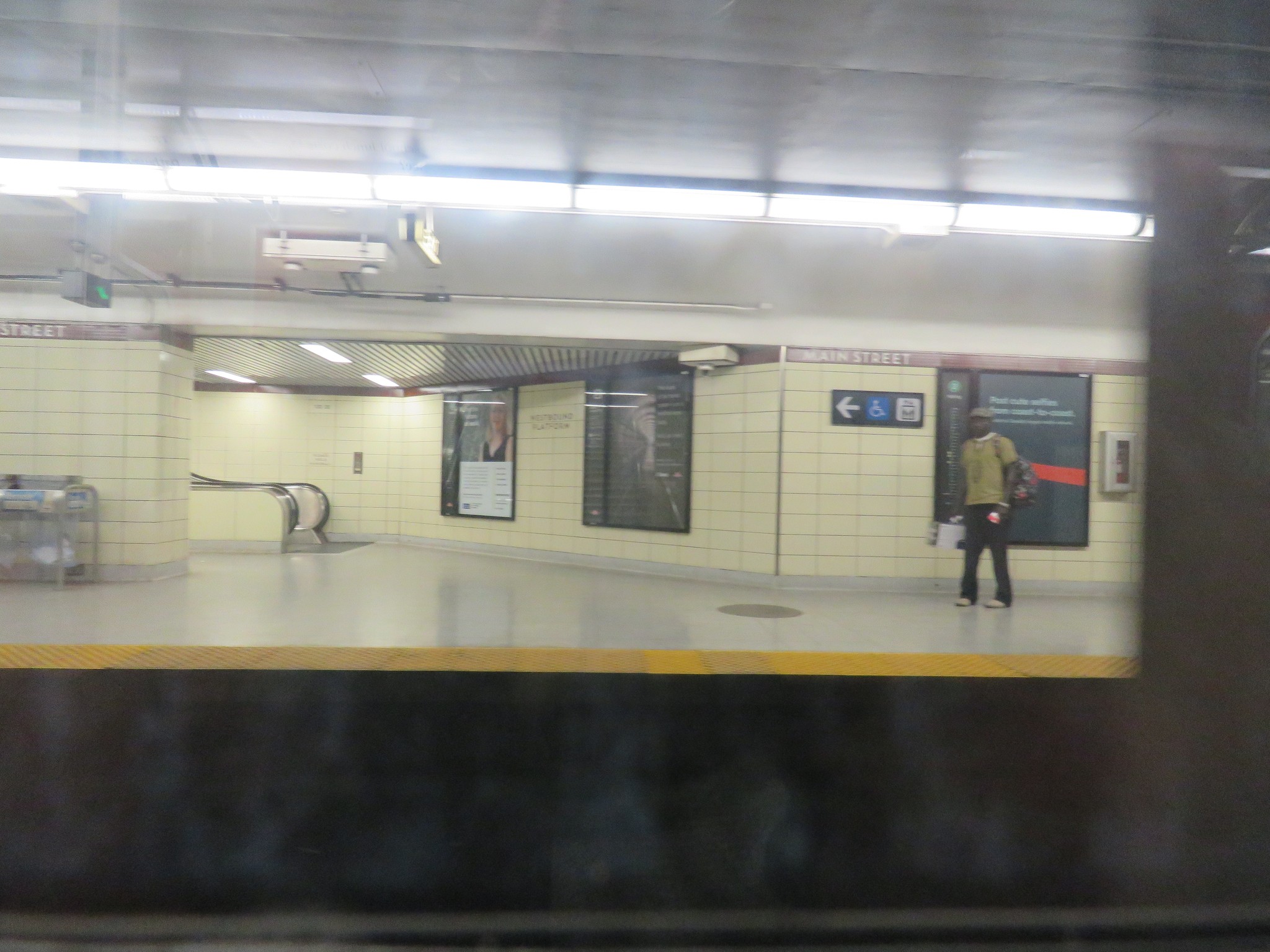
[969,408,995,419]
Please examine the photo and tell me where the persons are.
[956,407,1019,608]
[478,393,514,462]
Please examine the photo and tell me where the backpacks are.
[994,436,1040,508]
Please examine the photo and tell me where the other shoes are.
[955,597,974,605]
[984,598,1010,608]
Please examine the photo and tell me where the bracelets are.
[998,501,1012,511]
